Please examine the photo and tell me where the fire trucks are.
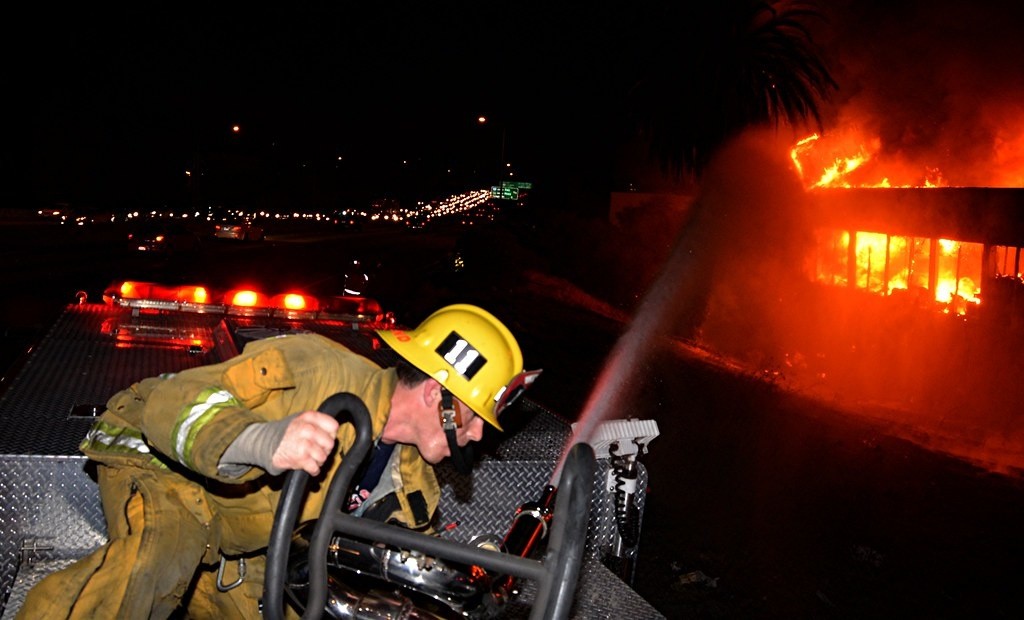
[0,274,684,620]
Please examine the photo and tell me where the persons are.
[12,304,525,620]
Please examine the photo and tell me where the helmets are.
[374,304,524,432]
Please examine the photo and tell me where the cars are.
[126,222,186,260]
[208,218,268,242]
